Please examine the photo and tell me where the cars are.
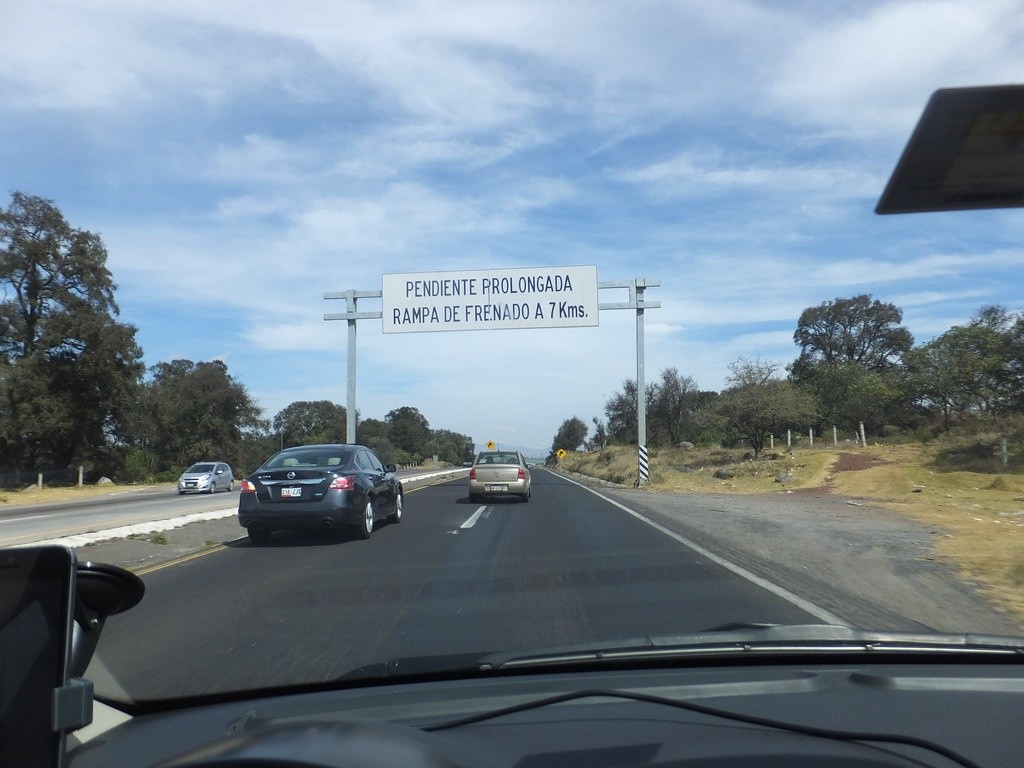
[238,443,404,542]
[468,450,534,503]
[177,460,235,495]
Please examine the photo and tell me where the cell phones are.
[0,542,77,768]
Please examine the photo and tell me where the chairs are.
[283,458,299,467]
[328,458,341,465]
